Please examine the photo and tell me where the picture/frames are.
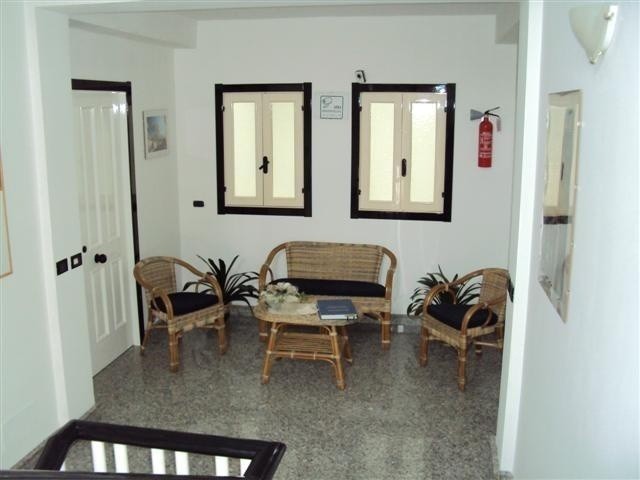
[0,153,14,280]
[141,107,172,161]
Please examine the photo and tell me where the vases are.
[265,298,298,309]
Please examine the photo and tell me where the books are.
[316,299,358,320]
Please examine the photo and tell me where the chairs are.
[417,267,509,392]
[131,254,228,373]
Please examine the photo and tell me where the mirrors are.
[535,89,585,325]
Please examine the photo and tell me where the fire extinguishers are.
[471,106,501,167]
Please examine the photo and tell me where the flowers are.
[261,283,304,300]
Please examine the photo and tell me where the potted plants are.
[179,254,261,323]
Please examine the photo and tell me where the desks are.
[253,303,363,391]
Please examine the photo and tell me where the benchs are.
[257,239,399,350]
